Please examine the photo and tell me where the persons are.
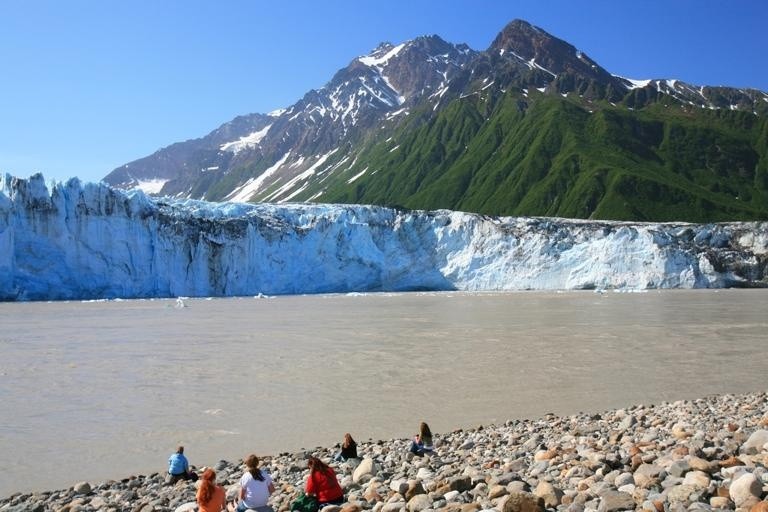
[193,468,228,512]
[305,456,345,509]
[228,454,277,512]
[167,444,196,480]
[410,421,433,458]
[333,432,358,462]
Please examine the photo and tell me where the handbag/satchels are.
[291,494,320,511]
